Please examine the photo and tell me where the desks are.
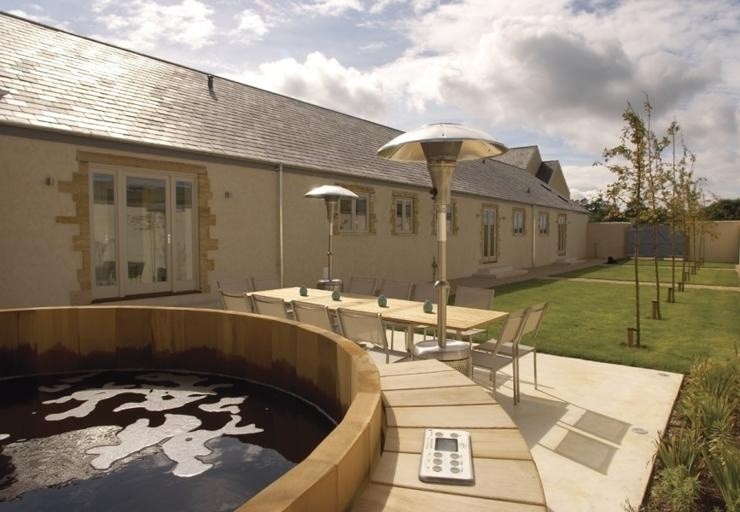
[248,285,510,359]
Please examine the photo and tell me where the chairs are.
[250,293,288,319]
[474,300,548,394]
[336,307,415,364]
[217,288,252,314]
[378,278,417,352]
[290,299,334,331]
[412,282,452,341]
[470,307,527,405]
[452,285,494,346]
[347,276,380,296]
